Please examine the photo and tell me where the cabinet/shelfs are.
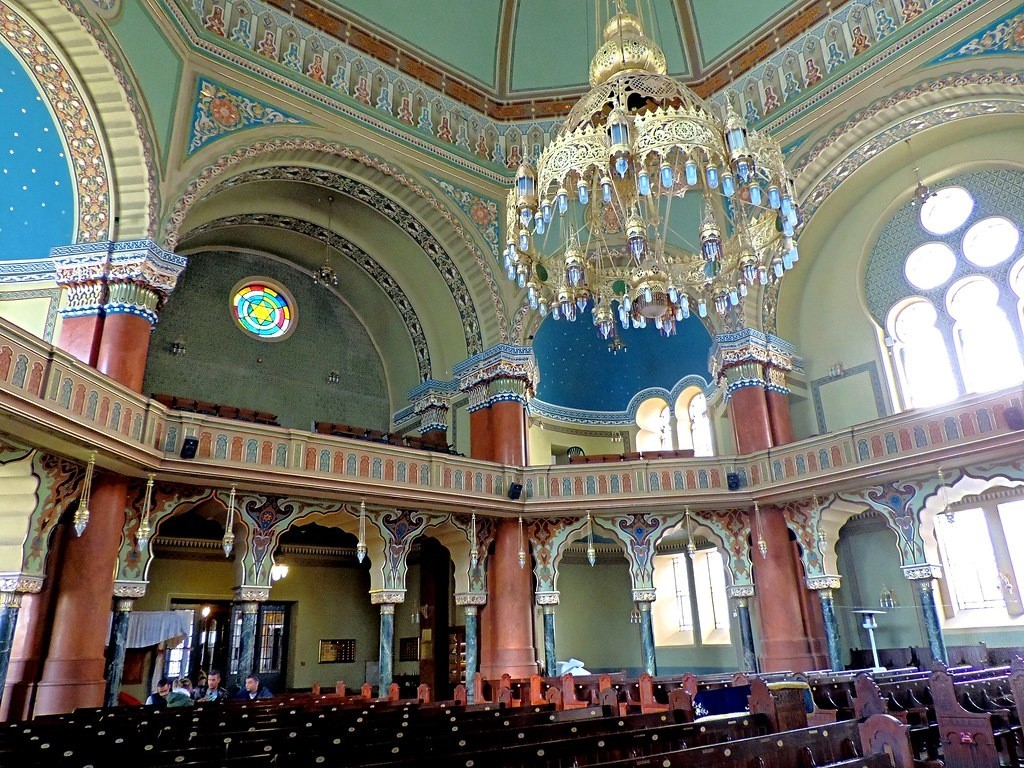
[449,626,466,699]
[392,675,419,699]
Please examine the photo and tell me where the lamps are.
[411,604,428,624]
[810,490,827,551]
[134,474,156,551]
[905,138,938,206]
[586,510,596,566]
[271,541,290,580]
[630,604,641,624]
[935,464,954,526]
[611,426,621,442]
[753,501,767,560]
[312,196,338,287]
[517,513,526,570]
[326,370,340,383]
[608,302,627,355]
[528,416,544,429]
[878,584,898,610]
[74,450,97,537]
[222,484,237,558]
[171,343,186,357]
[470,510,478,569]
[357,498,367,564]
[502,0,799,342]
[684,505,696,560]
[827,362,846,377]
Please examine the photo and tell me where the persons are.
[146,679,171,705]
[194,670,229,702]
[198,667,207,685]
[167,678,194,707]
[236,674,272,700]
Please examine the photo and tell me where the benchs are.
[0,655,1024,768]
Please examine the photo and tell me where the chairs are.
[569,449,695,464]
[151,392,281,428]
[567,446,586,464]
[314,421,464,458]
